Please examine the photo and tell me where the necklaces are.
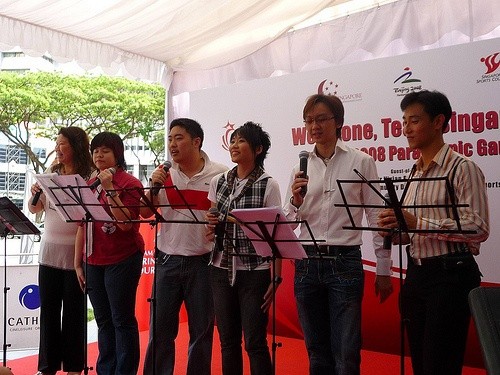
[317,152,334,163]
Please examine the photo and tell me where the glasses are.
[302,117,335,124]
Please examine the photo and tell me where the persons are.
[281,94,393,375]
[28,127,101,375]
[206,121,282,375]
[0,365,15,375]
[74,132,144,375]
[140,118,231,375]
[377,89,490,375]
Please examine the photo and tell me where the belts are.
[301,244,360,253]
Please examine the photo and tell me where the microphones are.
[209,207,225,251]
[299,151,310,197]
[152,161,172,195]
[383,193,392,250]
[32,187,42,206]
[89,167,116,191]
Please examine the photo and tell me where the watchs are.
[271,274,282,285]
